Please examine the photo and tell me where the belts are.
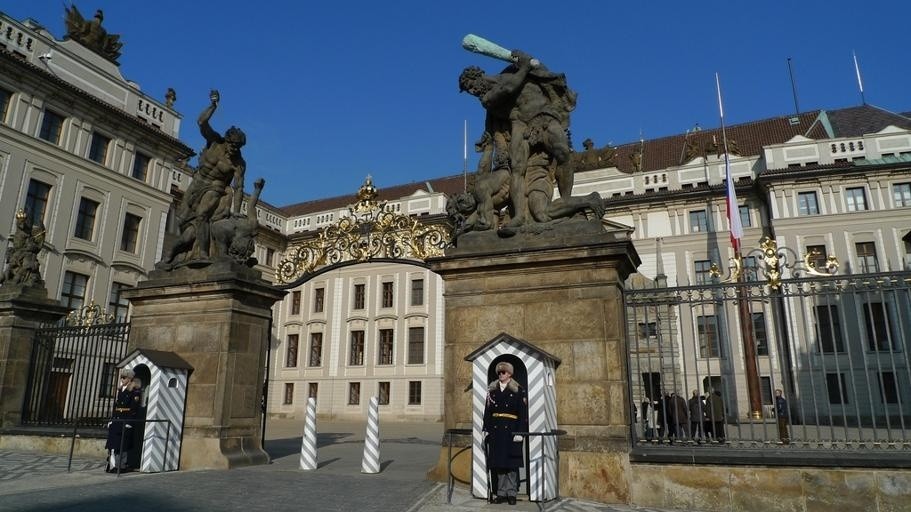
[492,412,517,420]
[114,407,131,412]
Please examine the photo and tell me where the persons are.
[640,385,726,444]
[482,359,529,506]
[633,399,639,424]
[447,51,607,233]
[771,388,791,443]
[104,369,145,473]
[161,87,268,268]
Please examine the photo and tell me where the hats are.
[496,362,515,375]
[119,367,136,379]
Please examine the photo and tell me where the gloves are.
[513,435,524,442]
[125,424,132,429]
[481,430,489,437]
[106,421,112,428]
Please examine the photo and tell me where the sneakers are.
[508,495,517,505]
[109,463,127,473]
[493,495,506,504]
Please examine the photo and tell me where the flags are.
[715,116,757,264]
